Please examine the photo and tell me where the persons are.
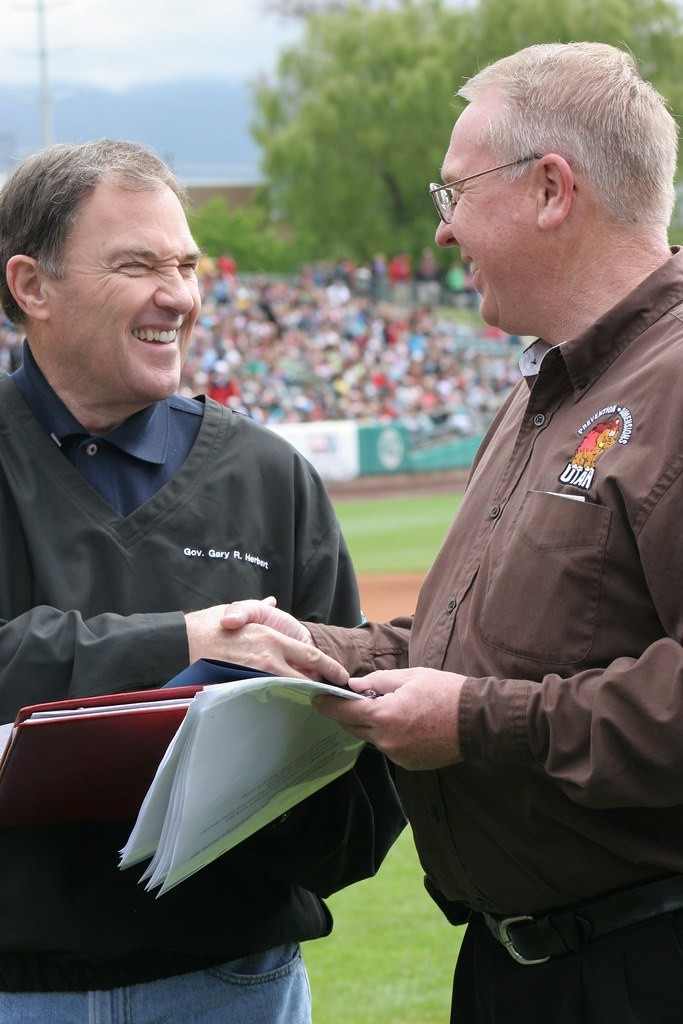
[221,40,683,1024]
[0,138,410,1024]
[0,247,546,439]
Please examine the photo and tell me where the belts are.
[483,871,683,966]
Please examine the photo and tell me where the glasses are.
[427,153,577,224]
